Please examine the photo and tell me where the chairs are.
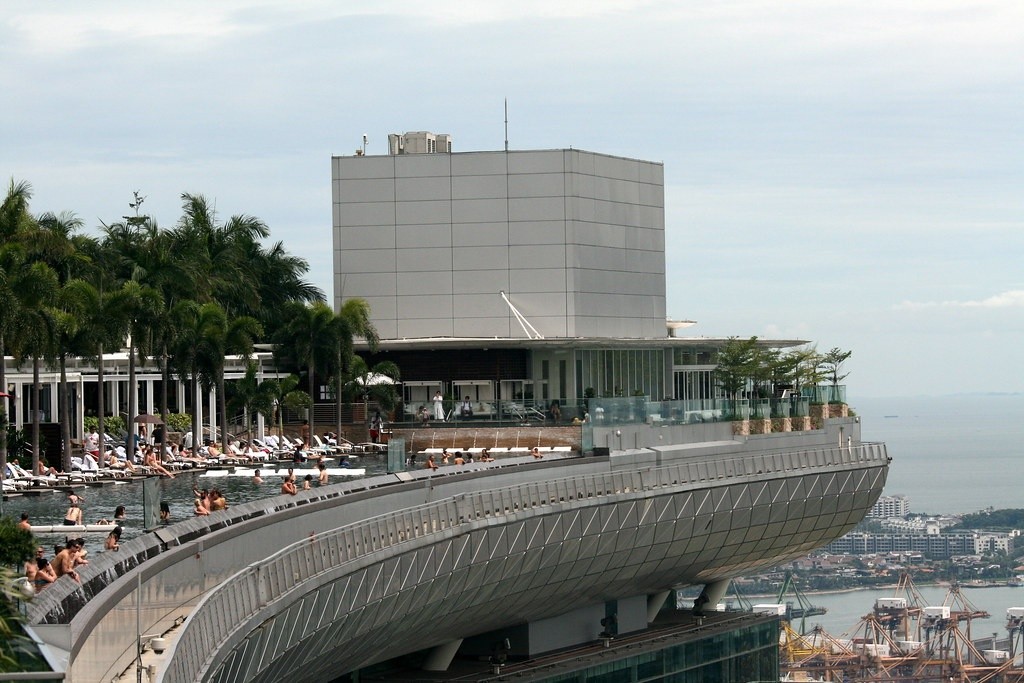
[0,431,376,494]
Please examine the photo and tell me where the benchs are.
[403,399,448,420]
[450,400,501,420]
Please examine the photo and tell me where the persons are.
[301,419,309,446]
[64,504,82,525]
[282,464,328,494]
[533,447,543,459]
[192,487,230,515]
[294,444,326,469]
[433,391,444,420]
[83,423,259,477]
[369,411,383,443]
[104,526,122,551]
[416,406,430,425]
[39,455,57,476]
[461,396,473,421]
[96,505,125,525]
[18,512,31,530]
[25,538,89,583]
[410,449,494,469]
[550,404,603,423]
[338,458,348,466]
[160,501,170,519]
[254,469,261,482]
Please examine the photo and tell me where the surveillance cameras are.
[150,638,166,654]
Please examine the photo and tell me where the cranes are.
[730,564,1024,683]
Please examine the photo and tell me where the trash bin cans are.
[381,428,393,444]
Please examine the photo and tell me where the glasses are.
[36,551,43,553]
[73,545,79,549]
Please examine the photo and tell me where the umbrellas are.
[134,414,165,442]
